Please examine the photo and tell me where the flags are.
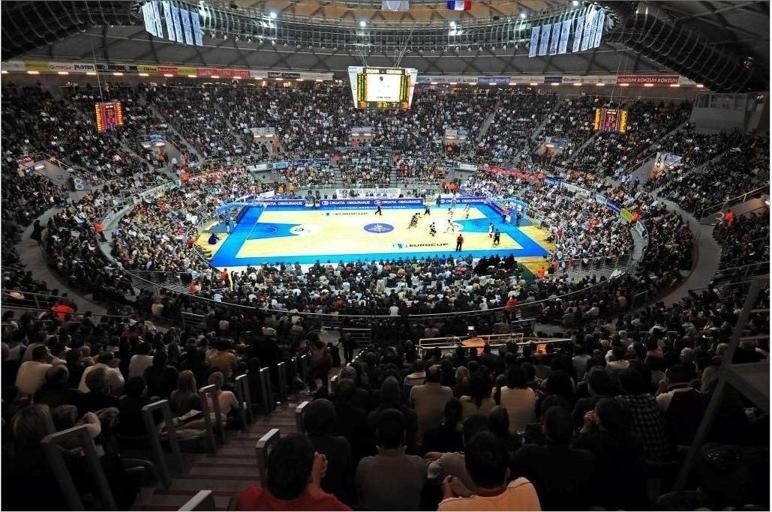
[381,0,410,13]
[446,0,472,12]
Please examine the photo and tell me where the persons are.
[428,222,437,236]
[450,196,457,208]
[488,221,495,239]
[375,205,383,216]
[2,73,769,206]
[502,207,509,224]
[442,219,455,234]
[517,212,522,227]
[233,251,770,510]
[414,212,422,228]
[526,201,769,267]
[456,234,464,250]
[1,202,233,511]
[425,203,432,216]
[494,229,500,243]
[448,209,455,220]
[408,213,418,230]
